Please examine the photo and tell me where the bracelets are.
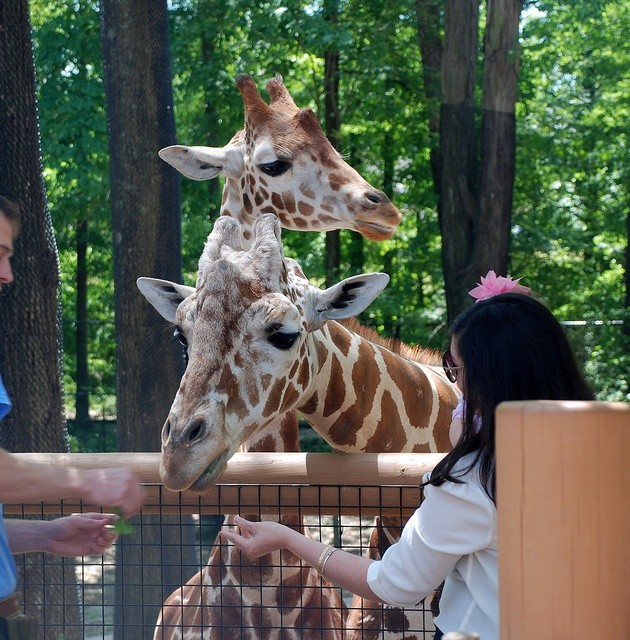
[317,546,332,577]
[321,547,339,583]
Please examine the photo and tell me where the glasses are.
[442,348,466,383]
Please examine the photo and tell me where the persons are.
[449,396,482,448]
[218,293,597,640]
[1,196,146,640]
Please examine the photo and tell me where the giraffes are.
[153,72,403,639]
[136,212,463,639]
